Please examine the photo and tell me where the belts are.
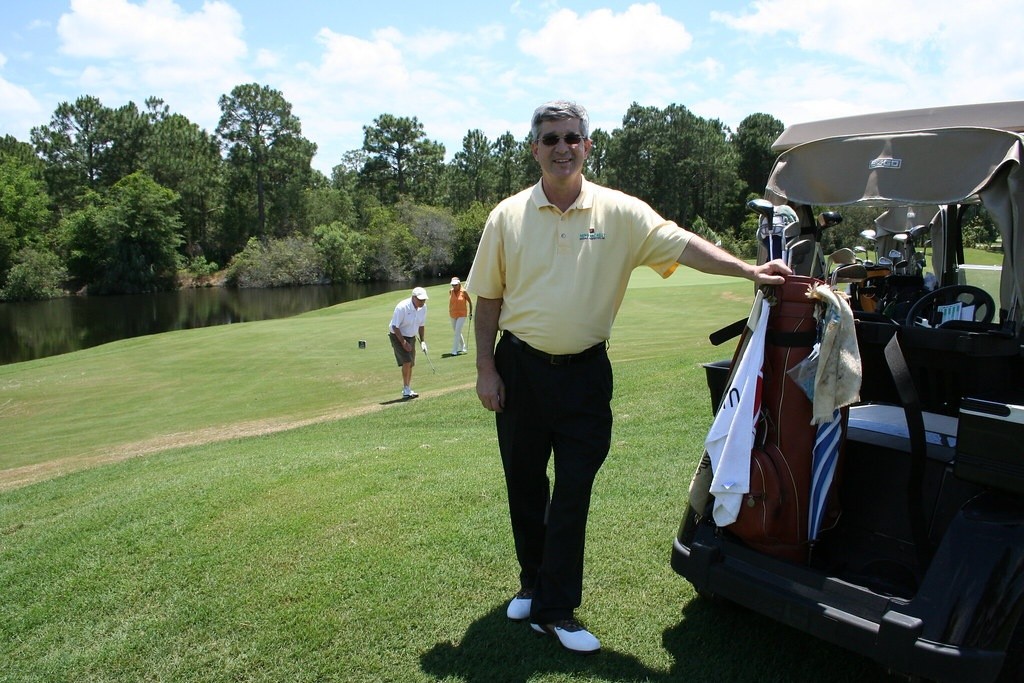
[503,330,606,365]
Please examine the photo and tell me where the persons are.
[464,99,794,653]
[447,276,473,355]
[388,287,428,398]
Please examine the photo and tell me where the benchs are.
[852,319,1024,419]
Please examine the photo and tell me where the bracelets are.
[469,313,473,316]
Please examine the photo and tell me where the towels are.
[702,299,771,527]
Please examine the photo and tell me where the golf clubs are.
[779,219,812,277]
[415,335,437,375]
[879,257,894,278]
[823,248,856,315]
[748,198,774,261]
[854,229,879,268]
[779,211,843,278]
[463,317,471,354]
[828,257,868,319]
[889,249,903,277]
[908,224,927,276]
[893,233,909,274]
[912,239,933,277]
[894,259,907,277]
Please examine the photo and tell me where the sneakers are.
[507,587,535,619]
[402,387,419,398]
[452,351,458,355]
[529,618,600,654]
[458,349,467,355]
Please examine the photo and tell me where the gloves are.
[420,342,427,353]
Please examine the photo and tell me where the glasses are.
[536,132,588,146]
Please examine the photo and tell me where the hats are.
[450,277,460,285]
[412,287,428,300]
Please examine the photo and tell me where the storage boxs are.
[950,395,1024,496]
